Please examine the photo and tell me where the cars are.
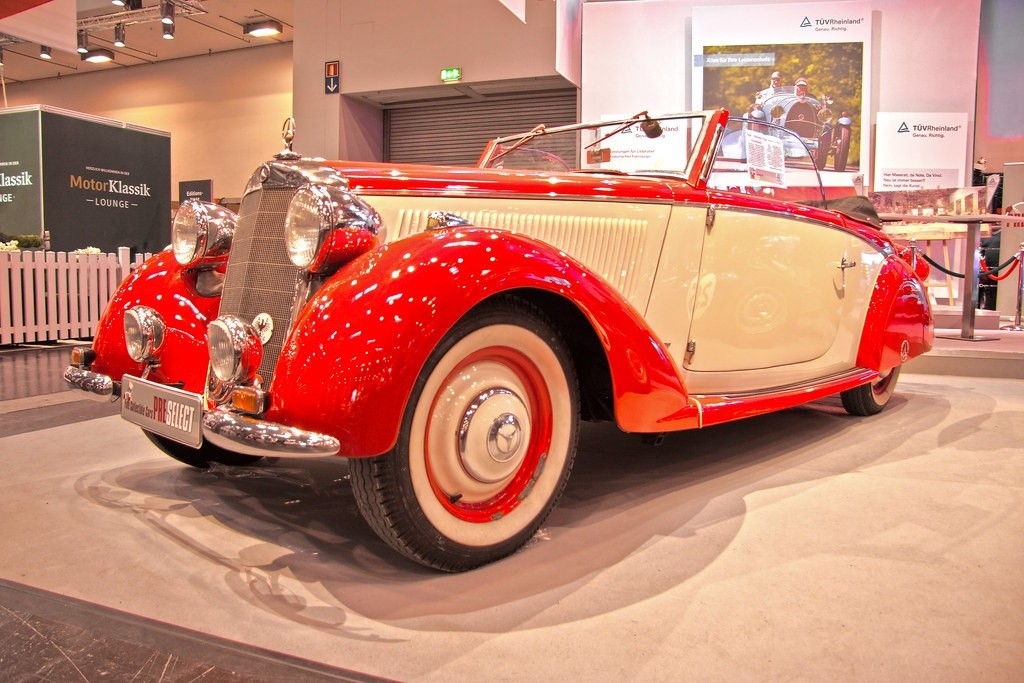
[65,108,940,574]
[741,85,850,170]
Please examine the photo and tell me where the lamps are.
[115,23,126,47]
[40,45,52,59]
[243,20,283,37]
[78,28,89,54]
[161,3,175,24]
[111,0,143,11]
[162,22,175,40]
[0,45,4,65]
[80,48,115,64]
